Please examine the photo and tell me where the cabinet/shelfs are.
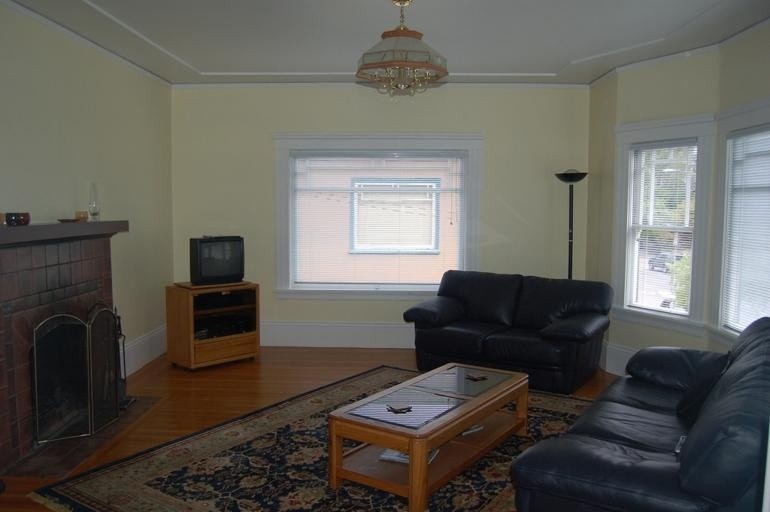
[164,282,262,370]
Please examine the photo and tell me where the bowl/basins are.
[5,212,30,225]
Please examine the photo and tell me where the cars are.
[648,252,683,273]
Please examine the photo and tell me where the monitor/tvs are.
[190,235,244,286]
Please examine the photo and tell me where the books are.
[461,424,485,436]
[379,446,440,465]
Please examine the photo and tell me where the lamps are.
[356,1,451,97]
[556,168,590,279]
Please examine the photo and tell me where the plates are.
[56,219,79,224]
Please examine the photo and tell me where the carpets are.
[24,366,594,510]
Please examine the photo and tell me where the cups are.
[75,208,88,222]
[87,199,100,222]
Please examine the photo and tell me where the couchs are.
[402,267,614,395]
[506,316,769,511]
